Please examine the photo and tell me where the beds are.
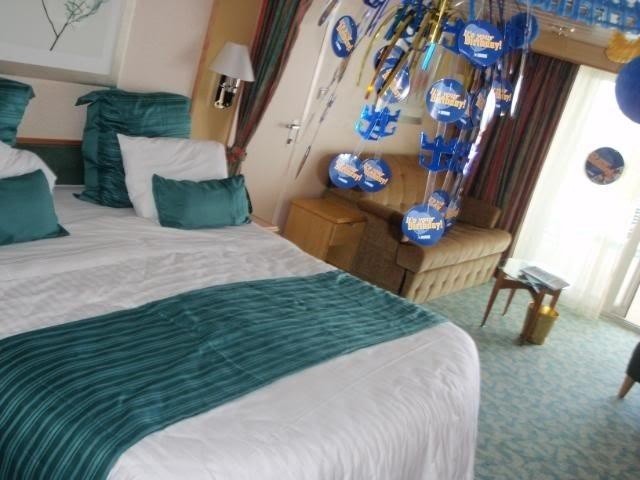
[0,138,482,480]
[480,258,565,345]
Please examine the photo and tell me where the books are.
[520,265,570,292]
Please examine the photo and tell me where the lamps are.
[208,42,256,110]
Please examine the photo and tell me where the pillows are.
[0,78,70,245]
[73,89,252,229]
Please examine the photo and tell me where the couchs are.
[317,153,511,304]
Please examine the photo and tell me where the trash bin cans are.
[522,303,559,345]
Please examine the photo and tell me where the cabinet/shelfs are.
[283,196,368,273]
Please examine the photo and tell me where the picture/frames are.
[0,0,136,89]
